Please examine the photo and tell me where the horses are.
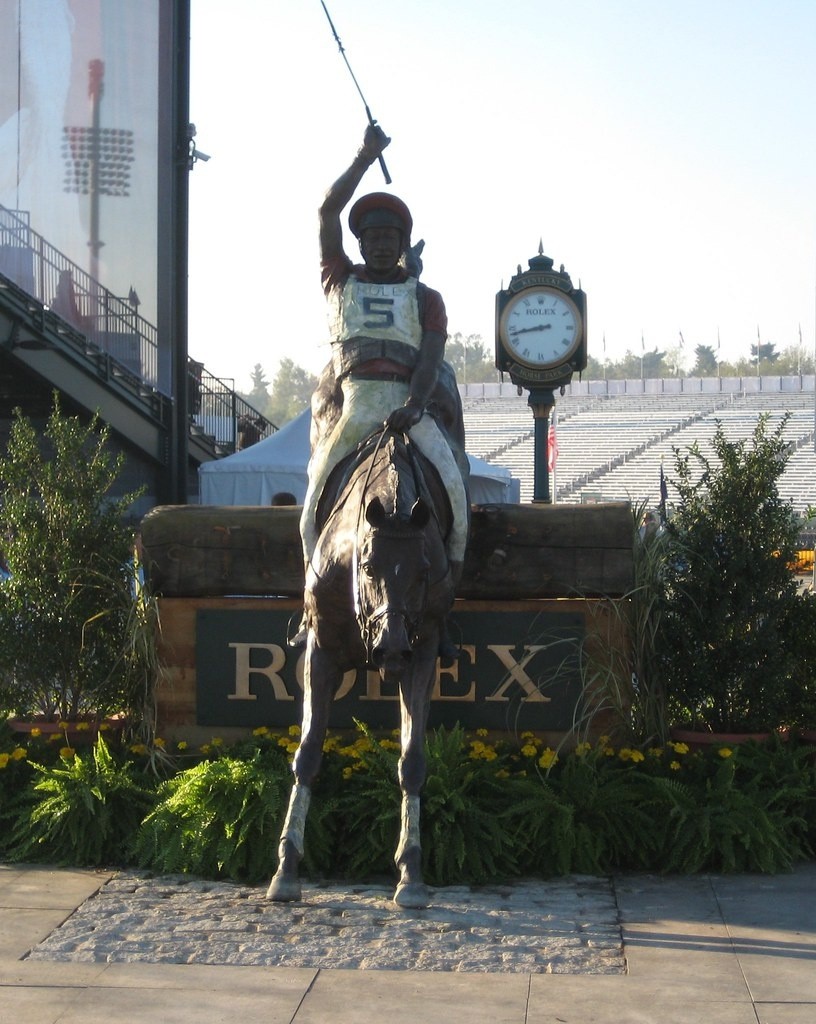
[263,239,470,910]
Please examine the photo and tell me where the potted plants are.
[629,414,816,763]
[0,387,161,748]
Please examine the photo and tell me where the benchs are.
[460,395,816,514]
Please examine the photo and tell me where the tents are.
[199,401,522,505]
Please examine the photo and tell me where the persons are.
[285,121,474,648]
[639,513,688,574]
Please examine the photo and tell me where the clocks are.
[499,286,583,369]
